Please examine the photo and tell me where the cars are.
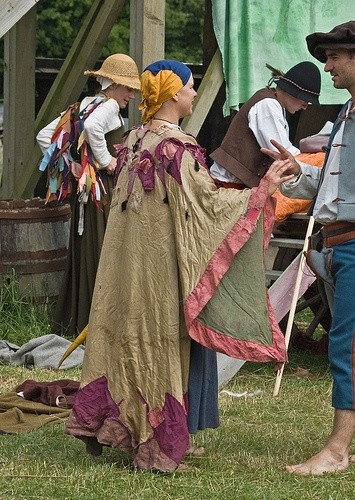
[0,57,213,201]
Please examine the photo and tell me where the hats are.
[84,53,141,90]
[305,21,355,63]
[273,61,321,107]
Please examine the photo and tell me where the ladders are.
[217,211,315,397]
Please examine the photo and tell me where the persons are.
[36,21,323,476]
[258,20,355,476]
